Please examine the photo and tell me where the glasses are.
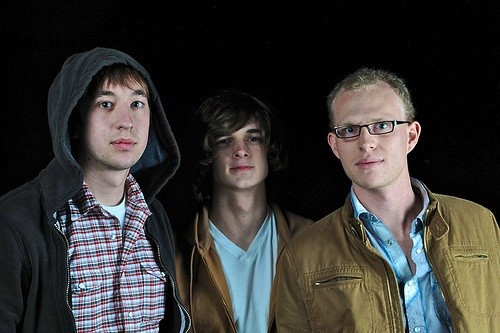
[333,120,411,137]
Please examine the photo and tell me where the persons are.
[0,47,191,333]
[171,90,317,333]
[276,67,500,333]
